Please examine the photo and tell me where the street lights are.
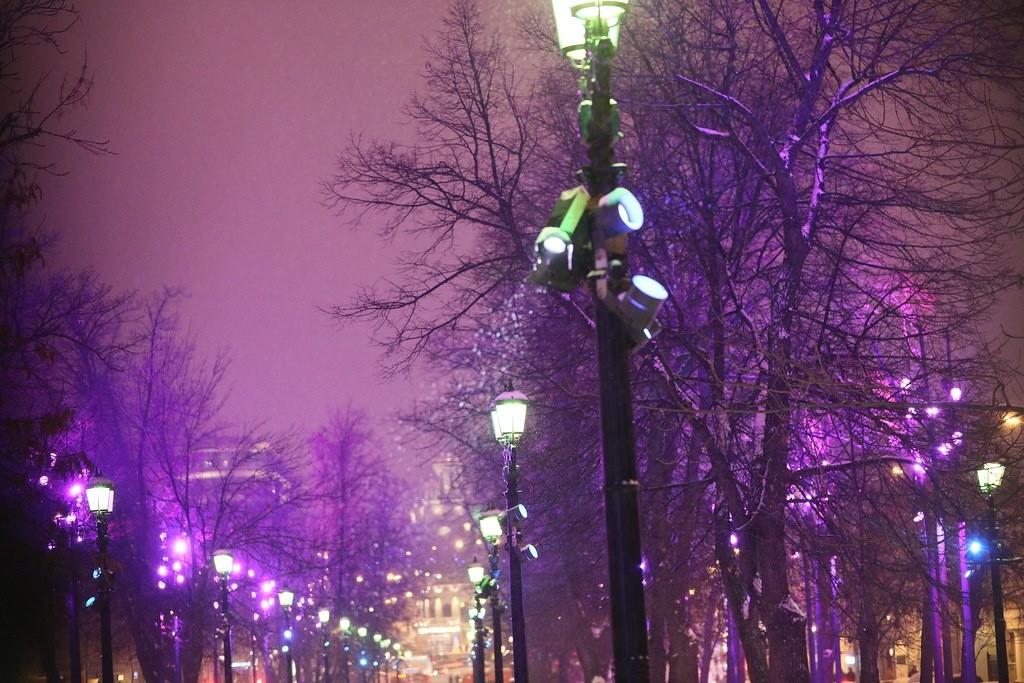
[382,636,390,683]
[394,642,400,683]
[489,376,538,683]
[468,557,489,683]
[976,458,1010,683]
[358,626,367,683]
[373,631,382,683]
[318,607,330,683]
[213,544,234,683]
[84,469,114,683]
[279,584,294,683]
[339,616,351,683]
[478,504,505,683]
[534,0,670,682]
